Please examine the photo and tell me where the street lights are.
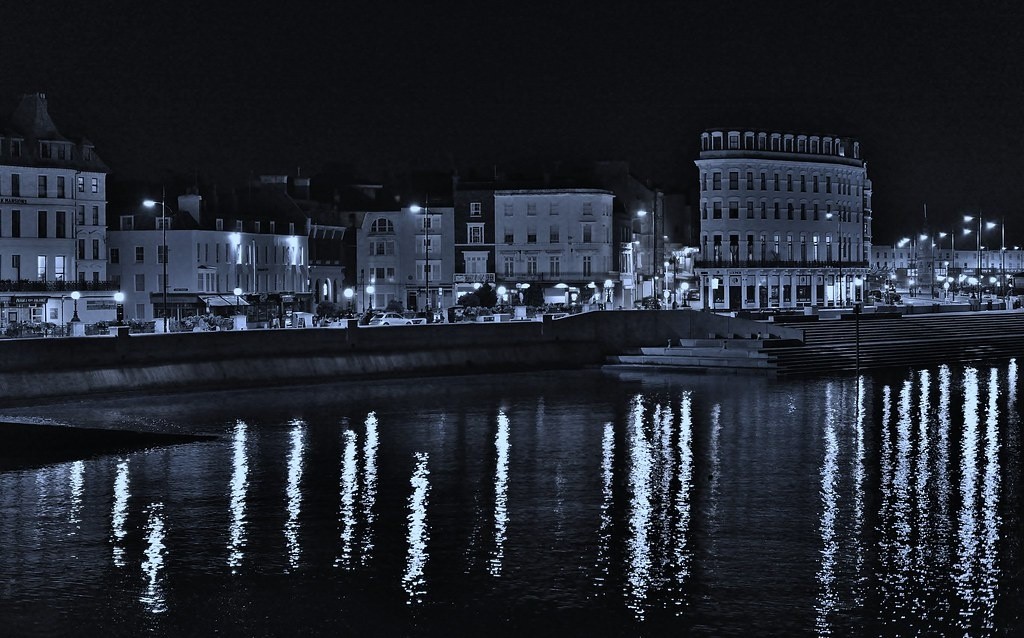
[665,262,669,311]
[940,232,954,301]
[411,205,430,322]
[638,210,656,308]
[897,236,916,298]
[988,222,1005,299]
[1002,248,1006,267]
[965,215,979,302]
[981,247,988,268]
[1015,246,1022,268]
[826,212,843,306]
[920,233,934,300]
[143,200,167,332]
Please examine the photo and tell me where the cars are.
[688,288,700,301]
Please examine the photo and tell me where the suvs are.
[369,312,413,325]
